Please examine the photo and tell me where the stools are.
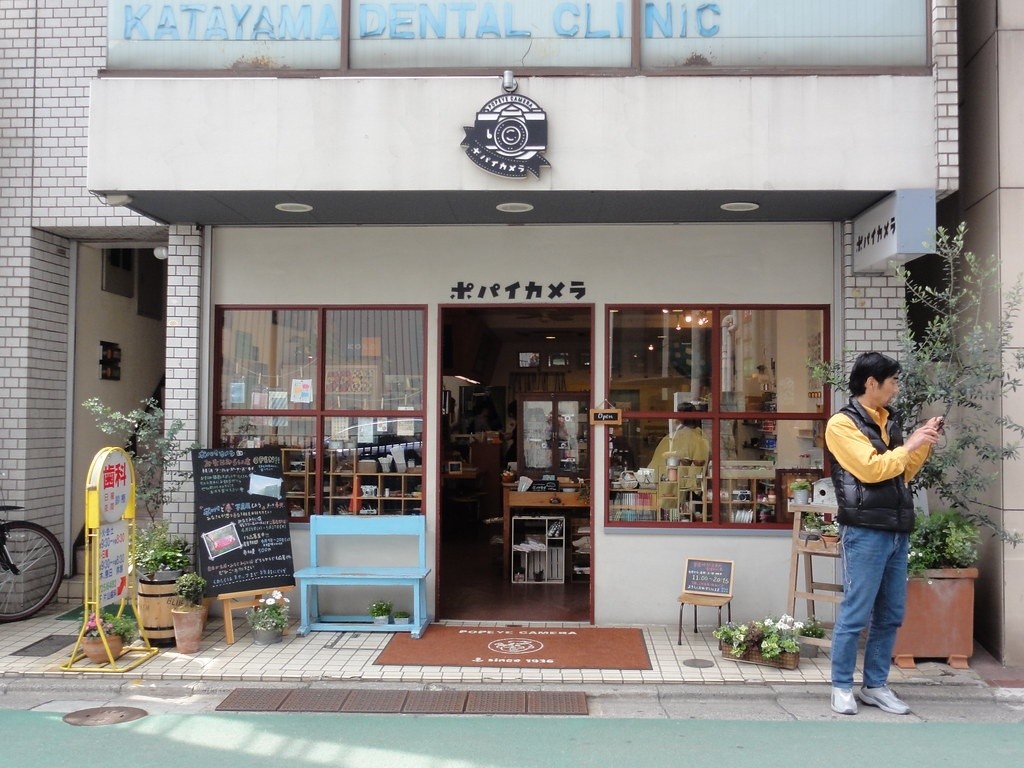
[447,478,488,542]
[678,592,733,649]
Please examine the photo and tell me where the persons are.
[647,402,710,511]
[824,352,945,717]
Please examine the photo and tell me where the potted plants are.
[804,515,825,536]
[171,572,208,654]
[789,480,812,504]
[393,610,411,624]
[129,520,194,649]
[80,396,205,582]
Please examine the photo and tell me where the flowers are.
[245,590,290,635]
[79,610,139,648]
[710,613,802,660]
[500,469,517,477]
[821,522,840,536]
[799,614,826,639]
[907,506,984,585]
[367,599,393,619]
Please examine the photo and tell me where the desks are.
[441,471,477,479]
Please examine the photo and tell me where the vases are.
[798,642,820,658]
[821,535,839,542]
[502,476,515,483]
[374,616,391,625]
[890,567,980,670]
[81,635,123,664]
[252,628,283,646]
[721,642,800,670]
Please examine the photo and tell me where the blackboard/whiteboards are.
[683,558,733,598]
[191,448,296,595]
[589,409,622,425]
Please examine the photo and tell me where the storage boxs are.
[742,423,760,450]
[707,459,776,478]
[290,462,423,517]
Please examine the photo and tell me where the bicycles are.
[0,505,65,623]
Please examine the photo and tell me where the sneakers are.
[830,687,858,715]
[858,684,910,714]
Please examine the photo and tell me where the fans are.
[514,309,576,323]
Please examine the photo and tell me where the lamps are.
[676,310,709,330]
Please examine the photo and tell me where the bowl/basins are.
[693,460,705,466]
[680,460,693,466]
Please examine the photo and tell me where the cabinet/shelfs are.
[502,481,591,586]
[452,441,504,521]
[610,488,657,511]
[705,476,775,524]
[657,465,705,522]
[787,503,869,648]
[281,448,423,518]
[99,340,121,380]
[517,389,590,482]
[757,389,778,486]
[511,516,566,585]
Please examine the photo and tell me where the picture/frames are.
[448,461,463,475]
[775,468,823,523]
[281,364,378,395]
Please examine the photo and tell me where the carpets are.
[371,623,654,671]
[55,603,136,621]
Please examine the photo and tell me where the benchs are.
[293,514,432,640]
